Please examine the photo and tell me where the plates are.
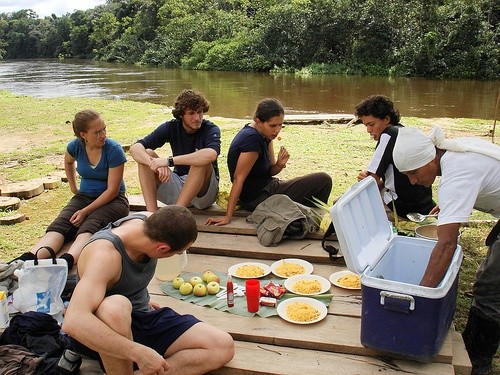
[228,262,272,279]
[283,274,331,296]
[276,296,328,325]
[271,258,313,279]
[329,270,362,290]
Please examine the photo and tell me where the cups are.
[245,279,260,313]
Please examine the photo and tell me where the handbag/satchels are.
[246,194,322,247]
[19,246,68,316]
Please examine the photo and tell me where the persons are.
[393,125,500,375]
[6,108,130,271]
[60,205,236,375]
[130,90,221,211]
[204,97,332,227]
[333,94,433,222]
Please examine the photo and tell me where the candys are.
[278,137,282,146]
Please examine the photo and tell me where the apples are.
[173,277,184,289]
[190,276,203,286]
[180,282,193,295]
[207,275,220,284]
[206,282,219,294]
[202,271,214,283]
[193,283,206,296]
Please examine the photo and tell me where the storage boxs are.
[260,297,277,307]
[328,176,464,362]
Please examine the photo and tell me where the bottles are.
[227,281,234,307]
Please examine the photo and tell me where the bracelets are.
[277,162,286,168]
[167,155,174,167]
